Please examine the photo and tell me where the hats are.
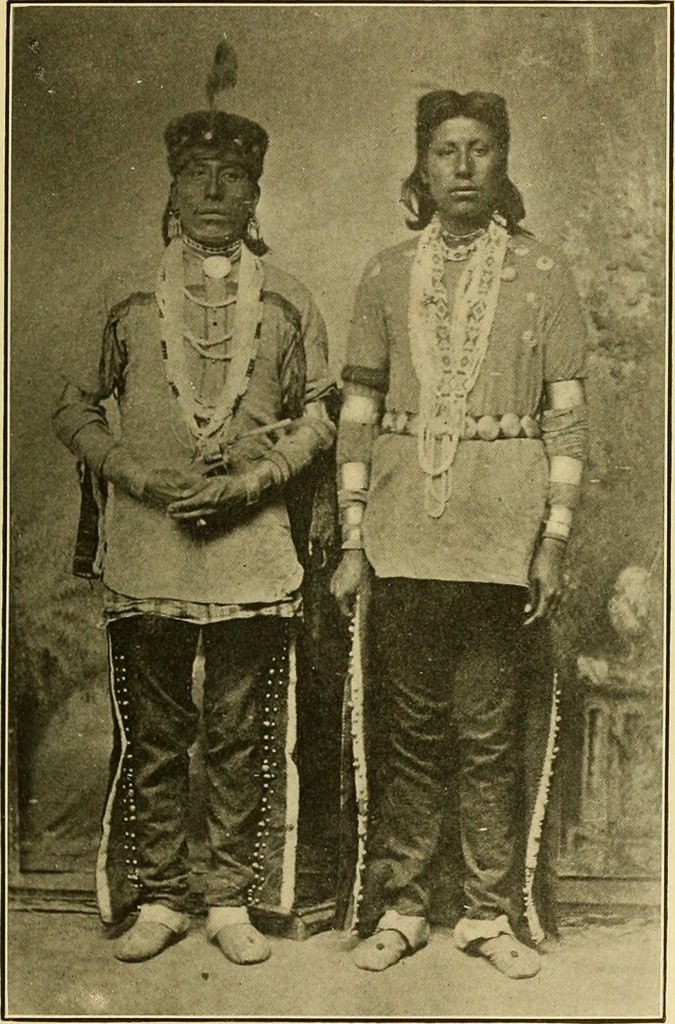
[164,40,268,182]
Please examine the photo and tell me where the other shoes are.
[353,909,429,971]
[114,903,191,962]
[453,914,541,979]
[207,904,271,965]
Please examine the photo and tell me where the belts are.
[379,408,543,443]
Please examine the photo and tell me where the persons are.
[329,88,590,980]
[50,107,335,965]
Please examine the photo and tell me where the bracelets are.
[238,471,261,506]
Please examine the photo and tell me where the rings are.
[196,517,207,527]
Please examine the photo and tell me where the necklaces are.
[154,236,262,447]
[407,211,506,519]
